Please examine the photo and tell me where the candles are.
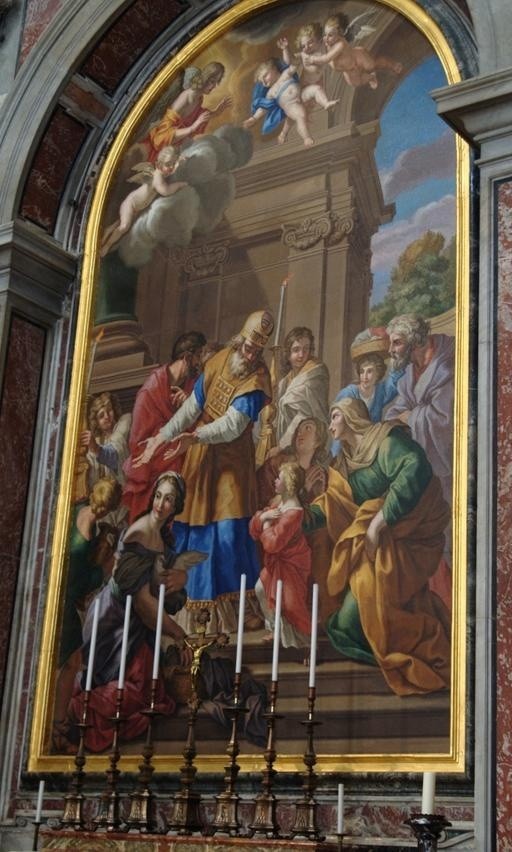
[151,583,165,679]
[337,783,343,833]
[308,584,320,689]
[271,579,284,680]
[84,598,102,690]
[234,573,246,673]
[35,780,43,822]
[116,595,132,690]
[421,769,437,815]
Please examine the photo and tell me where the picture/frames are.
[23,2,483,798]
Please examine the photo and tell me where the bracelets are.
[252,115,258,122]
[188,125,193,135]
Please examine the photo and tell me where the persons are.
[137,61,232,164]
[302,13,404,89]
[241,58,341,147]
[99,146,188,258]
[276,23,327,142]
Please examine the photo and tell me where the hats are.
[241,311,275,348]
